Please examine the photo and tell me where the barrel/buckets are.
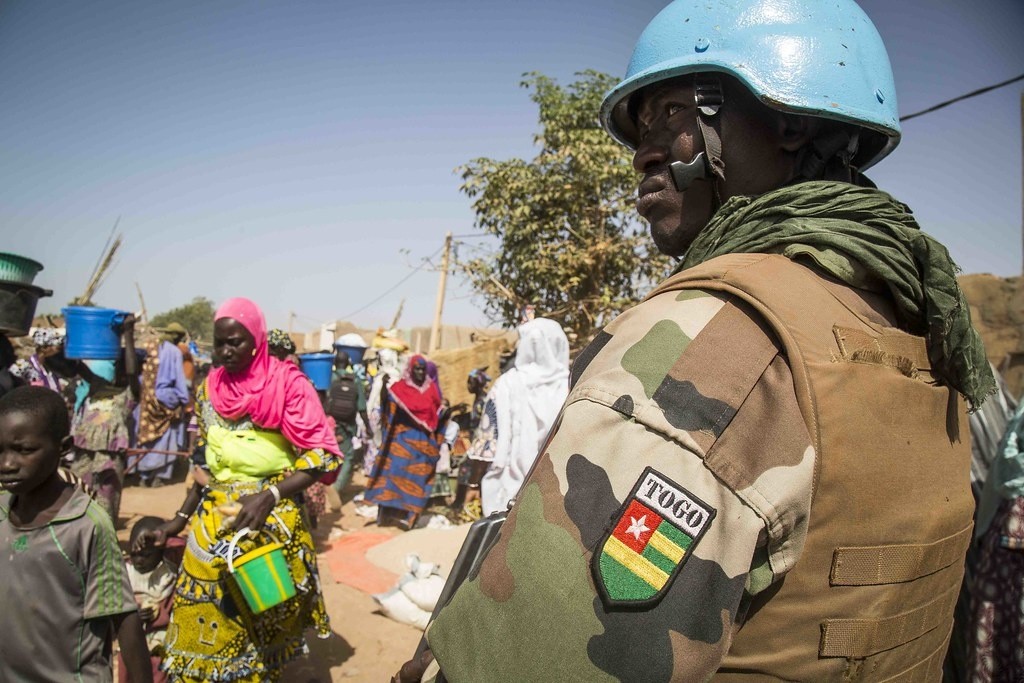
[334,343,368,365]
[229,527,297,612]
[62,306,129,361]
[300,352,335,389]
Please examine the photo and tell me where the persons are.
[452,366,493,507]
[465,317,570,517]
[267,328,326,528]
[125,322,210,488]
[154,297,345,683]
[68,313,141,528]
[422,0,999,683]
[326,351,374,510]
[363,354,469,531]
[354,349,400,475]
[0,326,66,395]
[0,386,153,683]
[945,360,1024,683]
[118,516,178,683]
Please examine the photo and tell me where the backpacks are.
[327,371,358,421]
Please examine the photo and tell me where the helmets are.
[598,0,901,173]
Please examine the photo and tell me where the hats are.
[469,366,491,386]
[32,328,62,346]
[267,329,292,349]
[158,322,186,333]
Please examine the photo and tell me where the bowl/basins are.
[0,280,53,336]
[0,252,44,283]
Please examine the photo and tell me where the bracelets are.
[267,485,280,504]
[466,483,479,488]
[176,511,191,522]
